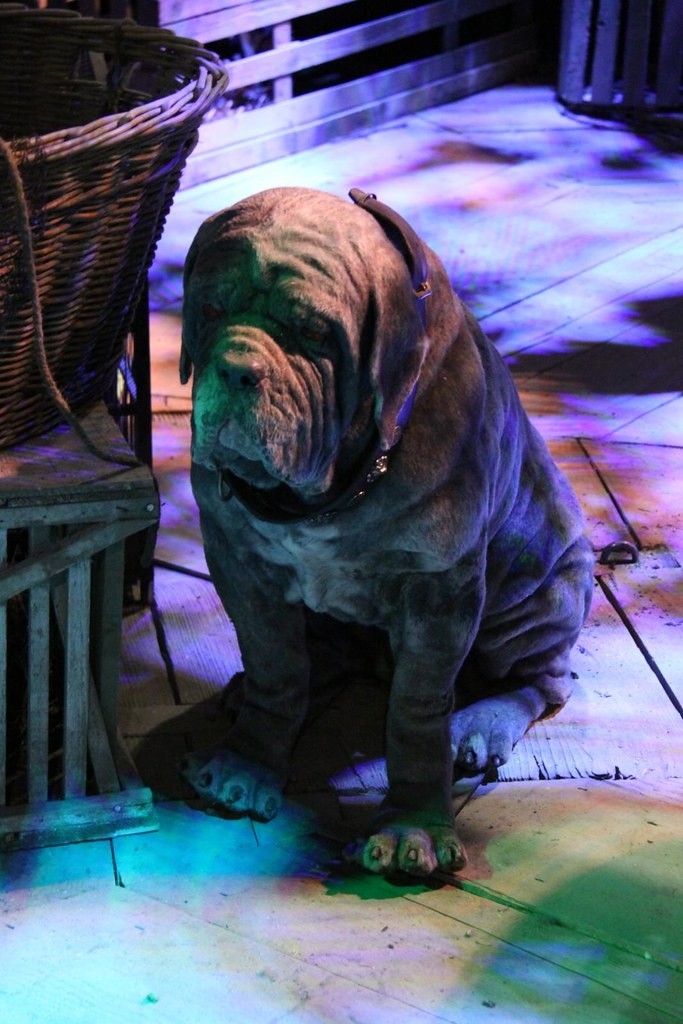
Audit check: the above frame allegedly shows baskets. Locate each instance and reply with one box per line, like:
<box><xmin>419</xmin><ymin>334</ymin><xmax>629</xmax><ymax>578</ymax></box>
<box><xmin>1</xmin><ymin>2</ymin><xmax>229</xmax><ymax>448</ymax></box>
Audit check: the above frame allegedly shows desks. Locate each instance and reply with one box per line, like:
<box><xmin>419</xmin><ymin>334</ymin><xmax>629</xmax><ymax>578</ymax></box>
<box><xmin>0</xmin><ymin>400</ymin><xmax>161</xmax><ymax>855</ymax></box>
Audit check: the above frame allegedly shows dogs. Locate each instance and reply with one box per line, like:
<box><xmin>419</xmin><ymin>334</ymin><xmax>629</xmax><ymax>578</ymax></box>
<box><xmin>119</xmin><ymin>185</ymin><xmax>592</xmax><ymax>872</ymax></box>
